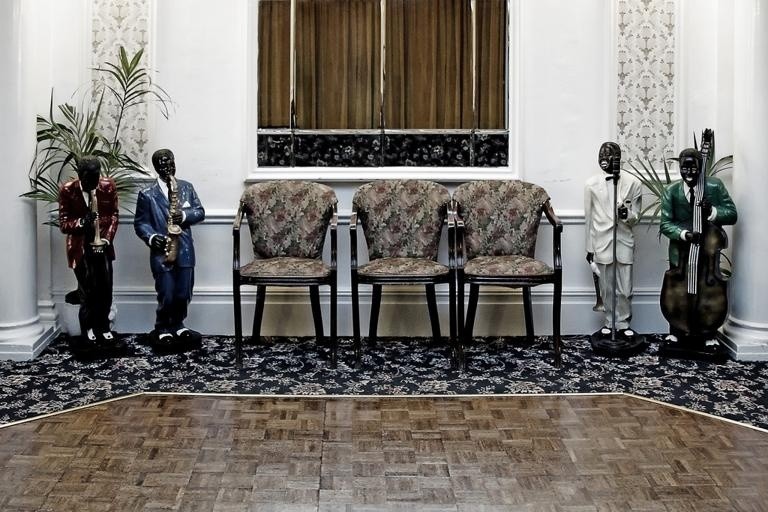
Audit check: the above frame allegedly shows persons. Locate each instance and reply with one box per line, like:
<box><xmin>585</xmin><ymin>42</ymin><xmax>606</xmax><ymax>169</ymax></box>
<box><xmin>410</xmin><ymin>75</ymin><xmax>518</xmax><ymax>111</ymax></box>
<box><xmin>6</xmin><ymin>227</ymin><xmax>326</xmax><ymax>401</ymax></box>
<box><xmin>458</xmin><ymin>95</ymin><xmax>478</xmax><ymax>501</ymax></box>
<box><xmin>134</xmin><ymin>148</ymin><xmax>206</xmax><ymax>345</ymax></box>
<box><xmin>658</xmin><ymin>149</ymin><xmax>739</xmax><ymax>353</ymax></box>
<box><xmin>57</xmin><ymin>155</ymin><xmax>121</xmax><ymax>345</ymax></box>
<box><xmin>583</xmin><ymin>142</ymin><xmax>644</xmax><ymax>345</ymax></box>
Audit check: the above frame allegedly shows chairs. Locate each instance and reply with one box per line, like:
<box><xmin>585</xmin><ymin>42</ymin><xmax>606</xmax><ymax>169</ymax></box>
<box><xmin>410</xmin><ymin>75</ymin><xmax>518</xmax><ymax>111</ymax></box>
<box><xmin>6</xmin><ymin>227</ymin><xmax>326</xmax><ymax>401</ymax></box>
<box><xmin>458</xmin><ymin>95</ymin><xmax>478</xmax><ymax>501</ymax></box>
<box><xmin>233</xmin><ymin>180</ymin><xmax>338</xmax><ymax>369</ymax></box>
<box><xmin>452</xmin><ymin>181</ymin><xmax>563</xmax><ymax>368</ymax></box>
<box><xmin>349</xmin><ymin>180</ymin><xmax>458</xmax><ymax>368</ymax></box>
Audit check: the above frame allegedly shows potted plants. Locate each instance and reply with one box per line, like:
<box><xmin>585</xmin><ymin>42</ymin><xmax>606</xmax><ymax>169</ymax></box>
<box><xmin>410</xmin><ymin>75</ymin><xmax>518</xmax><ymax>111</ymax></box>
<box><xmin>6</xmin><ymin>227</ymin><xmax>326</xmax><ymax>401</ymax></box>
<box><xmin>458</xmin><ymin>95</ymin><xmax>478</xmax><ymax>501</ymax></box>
<box><xmin>18</xmin><ymin>45</ymin><xmax>180</xmax><ymax>336</ymax></box>
